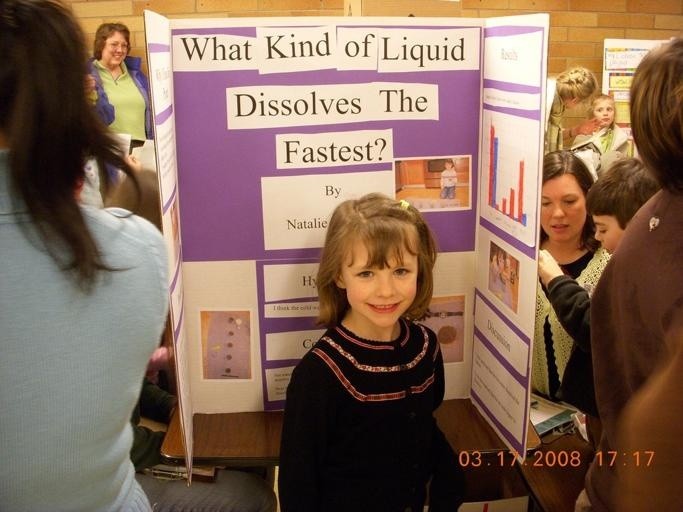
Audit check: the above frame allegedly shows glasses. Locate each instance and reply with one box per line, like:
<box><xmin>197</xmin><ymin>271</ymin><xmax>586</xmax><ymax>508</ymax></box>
<box><xmin>109</xmin><ymin>43</ymin><xmax>129</xmax><ymax>51</ymax></box>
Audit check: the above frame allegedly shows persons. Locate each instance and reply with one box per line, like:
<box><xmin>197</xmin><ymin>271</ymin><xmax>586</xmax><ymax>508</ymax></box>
<box><xmin>528</xmin><ymin>67</ymin><xmax>662</xmax><ymax>447</ymax></box>
<box><xmin>84</xmin><ymin>23</ymin><xmax>154</xmax><ymax>197</ymax></box>
<box><xmin>0</xmin><ymin>1</ymin><xmax>171</xmax><ymax>512</ymax></box>
<box><xmin>439</xmin><ymin>160</ymin><xmax>457</xmax><ymax>198</ymax></box>
<box><xmin>585</xmin><ymin>42</ymin><xmax>683</xmax><ymax>510</ymax></box>
<box><xmin>104</xmin><ymin>168</ymin><xmax>181</xmax><ymax>474</ymax></box>
<box><xmin>276</xmin><ymin>190</ymin><xmax>463</xmax><ymax>512</ymax></box>
